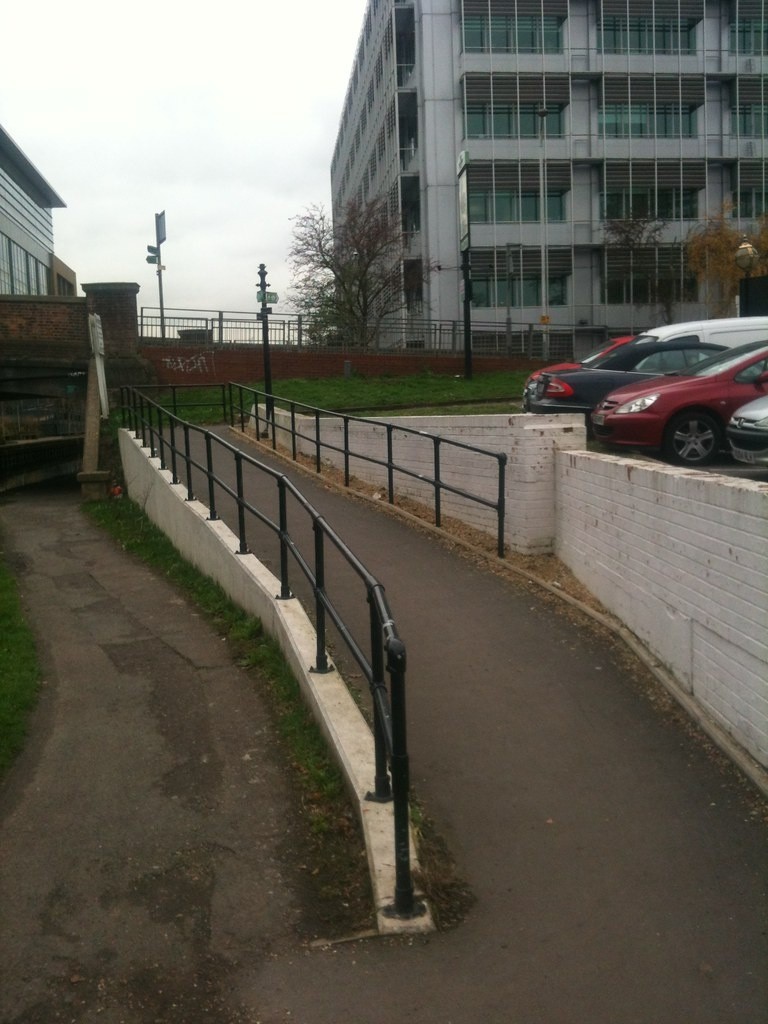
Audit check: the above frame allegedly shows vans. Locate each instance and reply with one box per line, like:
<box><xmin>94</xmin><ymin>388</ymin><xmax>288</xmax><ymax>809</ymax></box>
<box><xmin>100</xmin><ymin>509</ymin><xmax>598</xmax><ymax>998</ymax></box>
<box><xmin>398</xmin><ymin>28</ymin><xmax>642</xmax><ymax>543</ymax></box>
<box><xmin>635</xmin><ymin>317</ymin><xmax>768</xmax><ymax>378</ymax></box>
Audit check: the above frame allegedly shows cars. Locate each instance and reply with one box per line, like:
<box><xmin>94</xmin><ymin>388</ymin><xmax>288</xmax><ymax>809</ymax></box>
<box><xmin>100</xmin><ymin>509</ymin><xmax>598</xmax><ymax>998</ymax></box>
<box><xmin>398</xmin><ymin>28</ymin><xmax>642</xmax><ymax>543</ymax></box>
<box><xmin>591</xmin><ymin>340</ymin><xmax>768</xmax><ymax>467</ymax></box>
<box><xmin>525</xmin><ymin>334</ymin><xmax>637</xmax><ymax>388</ymax></box>
<box><xmin>729</xmin><ymin>394</ymin><xmax>768</xmax><ymax>472</ymax></box>
<box><xmin>527</xmin><ymin>341</ymin><xmax>736</xmax><ymax>441</ymax></box>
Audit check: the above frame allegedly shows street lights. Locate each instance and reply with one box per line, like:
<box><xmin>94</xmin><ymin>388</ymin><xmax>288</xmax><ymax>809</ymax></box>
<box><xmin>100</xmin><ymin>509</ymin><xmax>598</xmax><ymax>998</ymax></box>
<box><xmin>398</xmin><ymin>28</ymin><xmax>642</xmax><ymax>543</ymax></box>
<box><xmin>736</xmin><ymin>235</ymin><xmax>759</xmax><ymax>316</ymax></box>
